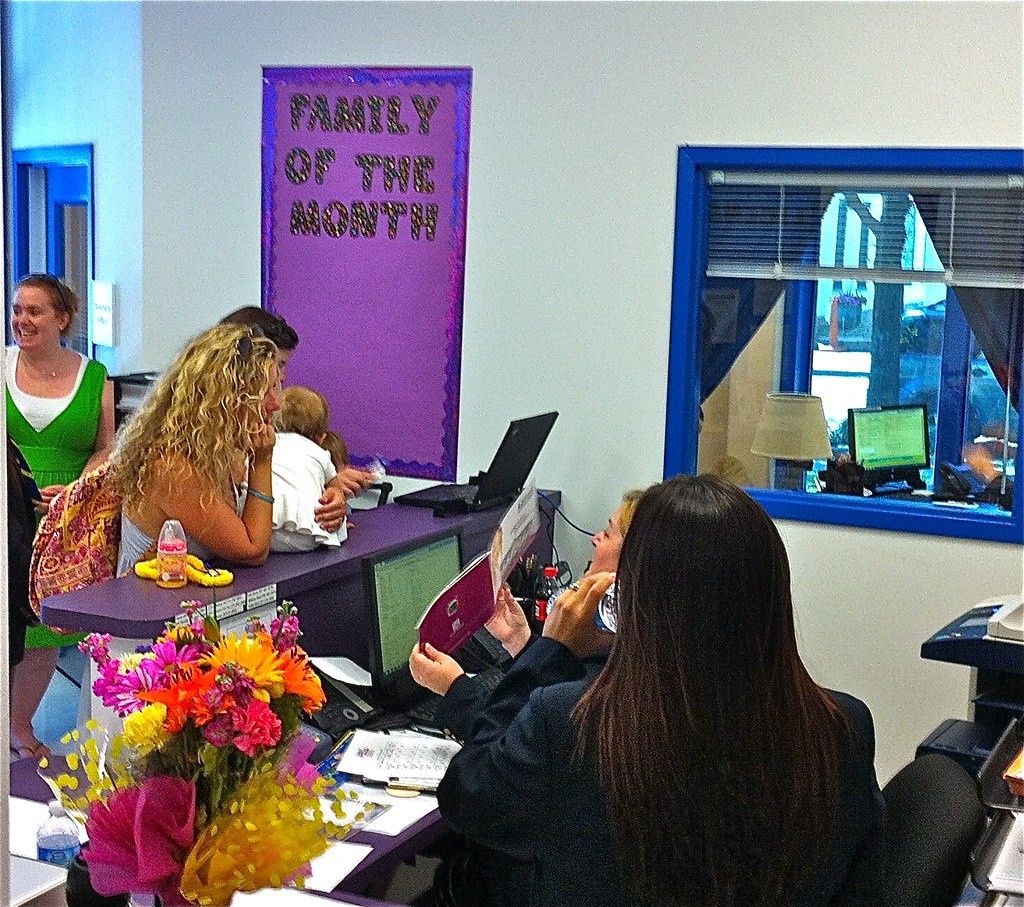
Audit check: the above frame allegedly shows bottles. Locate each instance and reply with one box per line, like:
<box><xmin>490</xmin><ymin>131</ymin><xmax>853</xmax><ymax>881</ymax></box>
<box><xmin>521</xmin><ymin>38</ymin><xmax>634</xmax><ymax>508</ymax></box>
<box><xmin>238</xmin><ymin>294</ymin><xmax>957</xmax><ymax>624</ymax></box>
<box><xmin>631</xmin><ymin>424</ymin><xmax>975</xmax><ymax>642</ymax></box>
<box><xmin>155</xmin><ymin>520</ymin><xmax>191</xmax><ymax>589</ymax></box>
<box><xmin>37</xmin><ymin>800</ymin><xmax>81</xmax><ymax>871</ymax></box>
<box><xmin>534</xmin><ymin>567</ymin><xmax>562</xmax><ymax>636</ymax></box>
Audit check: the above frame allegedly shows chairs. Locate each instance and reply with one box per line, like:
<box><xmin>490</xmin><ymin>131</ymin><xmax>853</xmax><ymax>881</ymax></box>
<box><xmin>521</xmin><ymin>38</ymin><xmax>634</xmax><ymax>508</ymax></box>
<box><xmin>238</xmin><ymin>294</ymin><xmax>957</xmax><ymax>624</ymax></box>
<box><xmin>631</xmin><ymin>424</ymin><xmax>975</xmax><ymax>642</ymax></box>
<box><xmin>825</xmin><ymin>750</ymin><xmax>987</xmax><ymax>907</ymax></box>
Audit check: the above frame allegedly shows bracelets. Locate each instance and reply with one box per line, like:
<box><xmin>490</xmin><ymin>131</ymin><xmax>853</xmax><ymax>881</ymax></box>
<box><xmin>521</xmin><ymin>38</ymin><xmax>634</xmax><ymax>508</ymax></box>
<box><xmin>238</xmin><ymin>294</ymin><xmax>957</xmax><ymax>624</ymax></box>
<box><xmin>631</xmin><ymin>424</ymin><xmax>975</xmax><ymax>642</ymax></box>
<box><xmin>247</xmin><ymin>489</ymin><xmax>274</xmax><ymax>503</ymax></box>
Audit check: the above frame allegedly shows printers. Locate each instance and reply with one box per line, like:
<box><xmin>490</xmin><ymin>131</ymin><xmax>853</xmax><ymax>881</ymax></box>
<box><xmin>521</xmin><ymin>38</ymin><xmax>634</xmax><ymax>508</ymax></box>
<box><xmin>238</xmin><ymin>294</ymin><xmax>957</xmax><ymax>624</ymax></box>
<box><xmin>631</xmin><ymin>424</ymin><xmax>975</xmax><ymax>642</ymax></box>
<box><xmin>920</xmin><ymin>592</ymin><xmax>1024</xmax><ymax>728</ymax></box>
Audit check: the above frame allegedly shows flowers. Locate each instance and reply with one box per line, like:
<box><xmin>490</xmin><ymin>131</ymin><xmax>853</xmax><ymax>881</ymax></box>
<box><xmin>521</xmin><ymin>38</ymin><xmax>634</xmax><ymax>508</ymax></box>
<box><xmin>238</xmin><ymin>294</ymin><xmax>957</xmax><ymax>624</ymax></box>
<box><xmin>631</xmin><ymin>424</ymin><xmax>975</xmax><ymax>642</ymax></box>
<box><xmin>37</xmin><ymin>598</ymin><xmax>378</xmax><ymax>907</ymax></box>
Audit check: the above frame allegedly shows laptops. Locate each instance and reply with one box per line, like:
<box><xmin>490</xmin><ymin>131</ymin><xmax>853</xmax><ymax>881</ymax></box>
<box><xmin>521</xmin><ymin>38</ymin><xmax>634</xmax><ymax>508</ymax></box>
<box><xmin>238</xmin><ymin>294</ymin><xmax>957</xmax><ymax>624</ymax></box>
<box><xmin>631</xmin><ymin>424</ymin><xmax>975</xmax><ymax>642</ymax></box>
<box><xmin>393</xmin><ymin>411</ymin><xmax>560</xmax><ymax>512</ymax></box>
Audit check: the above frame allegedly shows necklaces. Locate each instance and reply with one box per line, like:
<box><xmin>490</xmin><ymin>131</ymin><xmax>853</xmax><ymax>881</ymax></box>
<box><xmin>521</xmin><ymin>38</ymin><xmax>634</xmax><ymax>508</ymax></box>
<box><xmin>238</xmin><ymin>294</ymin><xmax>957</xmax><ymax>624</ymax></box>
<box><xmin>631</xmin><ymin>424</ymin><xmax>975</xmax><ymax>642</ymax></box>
<box><xmin>22</xmin><ymin>354</ymin><xmax>58</xmax><ymax>378</ymax></box>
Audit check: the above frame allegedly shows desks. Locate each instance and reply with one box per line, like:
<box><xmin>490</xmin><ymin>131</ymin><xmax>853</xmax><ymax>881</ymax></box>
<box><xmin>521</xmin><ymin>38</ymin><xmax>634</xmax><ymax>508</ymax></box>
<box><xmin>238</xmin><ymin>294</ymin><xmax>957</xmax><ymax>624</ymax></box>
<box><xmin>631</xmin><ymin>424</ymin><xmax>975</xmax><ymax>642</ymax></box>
<box><xmin>6</xmin><ymin>488</ymin><xmax>563</xmax><ymax>907</ymax></box>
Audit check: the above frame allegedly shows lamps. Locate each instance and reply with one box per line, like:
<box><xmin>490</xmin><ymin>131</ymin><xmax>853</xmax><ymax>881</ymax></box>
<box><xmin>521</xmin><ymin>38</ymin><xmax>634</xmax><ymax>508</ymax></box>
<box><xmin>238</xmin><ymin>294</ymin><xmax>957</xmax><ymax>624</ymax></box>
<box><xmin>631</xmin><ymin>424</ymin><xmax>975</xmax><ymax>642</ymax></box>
<box><xmin>749</xmin><ymin>392</ymin><xmax>833</xmax><ymax>492</ymax></box>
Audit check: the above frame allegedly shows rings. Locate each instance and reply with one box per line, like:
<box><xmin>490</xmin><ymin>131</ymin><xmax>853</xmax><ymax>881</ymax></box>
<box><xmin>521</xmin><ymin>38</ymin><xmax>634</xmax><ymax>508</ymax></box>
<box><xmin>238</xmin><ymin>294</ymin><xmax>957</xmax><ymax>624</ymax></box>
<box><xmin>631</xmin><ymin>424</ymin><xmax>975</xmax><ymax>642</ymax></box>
<box><xmin>569</xmin><ymin>582</ymin><xmax>580</xmax><ymax>590</ymax></box>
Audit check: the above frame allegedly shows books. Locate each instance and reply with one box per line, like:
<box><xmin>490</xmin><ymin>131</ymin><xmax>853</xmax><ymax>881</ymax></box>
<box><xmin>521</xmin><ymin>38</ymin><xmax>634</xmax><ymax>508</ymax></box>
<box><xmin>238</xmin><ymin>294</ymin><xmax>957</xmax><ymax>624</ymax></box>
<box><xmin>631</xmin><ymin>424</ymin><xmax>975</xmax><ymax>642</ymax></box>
<box><xmin>414</xmin><ymin>475</ymin><xmax>543</xmax><ymax>662</ymax></box>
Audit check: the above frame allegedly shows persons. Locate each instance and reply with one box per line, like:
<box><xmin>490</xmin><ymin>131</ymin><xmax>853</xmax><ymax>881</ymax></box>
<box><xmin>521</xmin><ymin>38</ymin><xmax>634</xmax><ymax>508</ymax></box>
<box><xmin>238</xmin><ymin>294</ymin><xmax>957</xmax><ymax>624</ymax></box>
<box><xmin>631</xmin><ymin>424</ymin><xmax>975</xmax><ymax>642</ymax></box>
<box><xmin>490</xmin><ymin>528</ymin><xmax>503</xmax><ymax>600</ymax></box>
<box><xmin>74</xmin><ymin>305</ymin><xmax>381</xmax><ymax>760</ymax></box>
<box><xmin>963</xmin><ymin>419</ymin><xmax>1018</xmax><ymax>513</ymax></box>
<box><xmin>8</xmin><ymin>273</ymin><xmax>116</xmax><ymax>759</ymax></box>
<box><xmin>434</xmin><ymin>470</ymin><xmax>889</xmax><ymax>907</ymax></box>
<box><xmin>406</xmin><ymin>488</ymin><xmax>647</xmax><ymax>747</ymax></box>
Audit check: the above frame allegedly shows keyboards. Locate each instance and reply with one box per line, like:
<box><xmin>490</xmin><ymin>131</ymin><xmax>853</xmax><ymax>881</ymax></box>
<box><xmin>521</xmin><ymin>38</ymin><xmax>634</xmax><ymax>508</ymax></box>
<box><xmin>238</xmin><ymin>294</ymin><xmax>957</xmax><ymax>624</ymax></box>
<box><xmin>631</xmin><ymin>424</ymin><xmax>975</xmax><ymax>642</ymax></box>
<box><xmin>405</xmin><ymin>668</ymin><xmax>506</xmax><ymax>726</ymax></box>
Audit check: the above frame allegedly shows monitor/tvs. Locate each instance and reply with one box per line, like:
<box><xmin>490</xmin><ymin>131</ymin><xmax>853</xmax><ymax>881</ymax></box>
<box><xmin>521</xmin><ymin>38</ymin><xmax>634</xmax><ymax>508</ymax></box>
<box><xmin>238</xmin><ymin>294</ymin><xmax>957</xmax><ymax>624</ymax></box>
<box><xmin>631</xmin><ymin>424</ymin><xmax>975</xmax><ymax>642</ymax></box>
<box><xmin>849</xmin><ymin>405</ymin><xmax>930</xmax><ymax>492</ymax></box>
<box><xmin>361</xmin><ymin>523</ymin><xmax>467</xmax><ymax>708</ymax></box>
